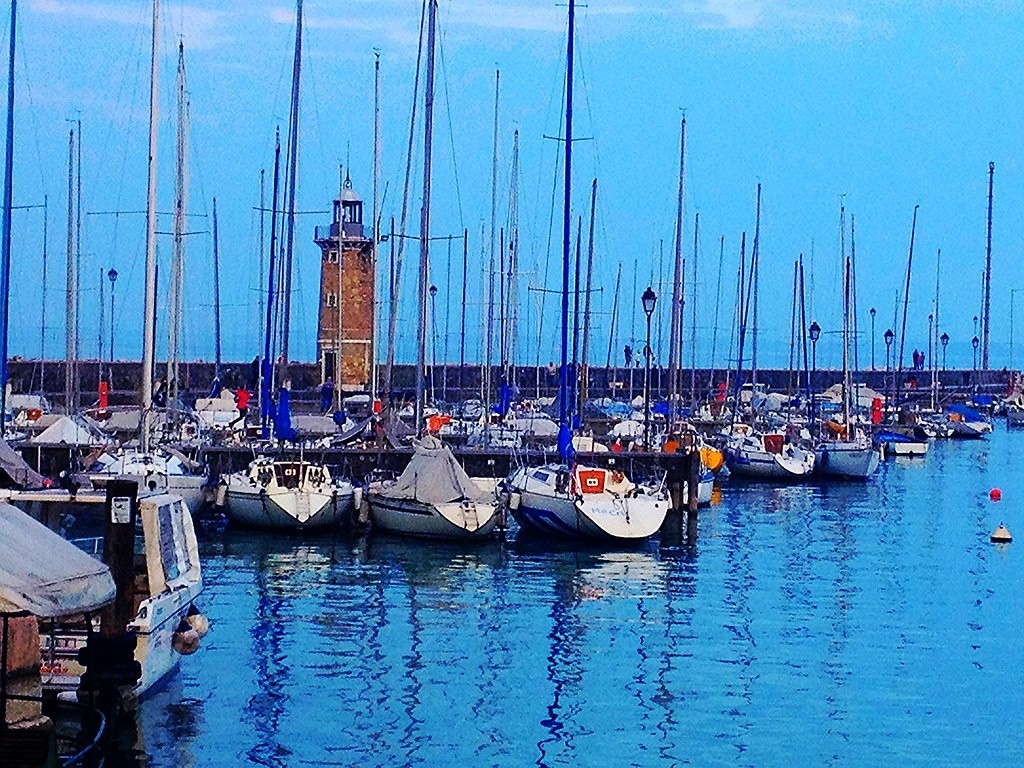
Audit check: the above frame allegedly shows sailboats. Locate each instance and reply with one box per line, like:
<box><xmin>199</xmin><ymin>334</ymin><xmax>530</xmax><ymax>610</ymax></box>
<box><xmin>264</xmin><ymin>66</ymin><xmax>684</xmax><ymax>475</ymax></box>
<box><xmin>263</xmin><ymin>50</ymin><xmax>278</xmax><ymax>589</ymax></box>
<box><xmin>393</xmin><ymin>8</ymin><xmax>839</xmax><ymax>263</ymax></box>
<box><xmin>2</xmin><ymin>0</ymin><xmax>1023</xmax><ymax>717</ymax></box>
